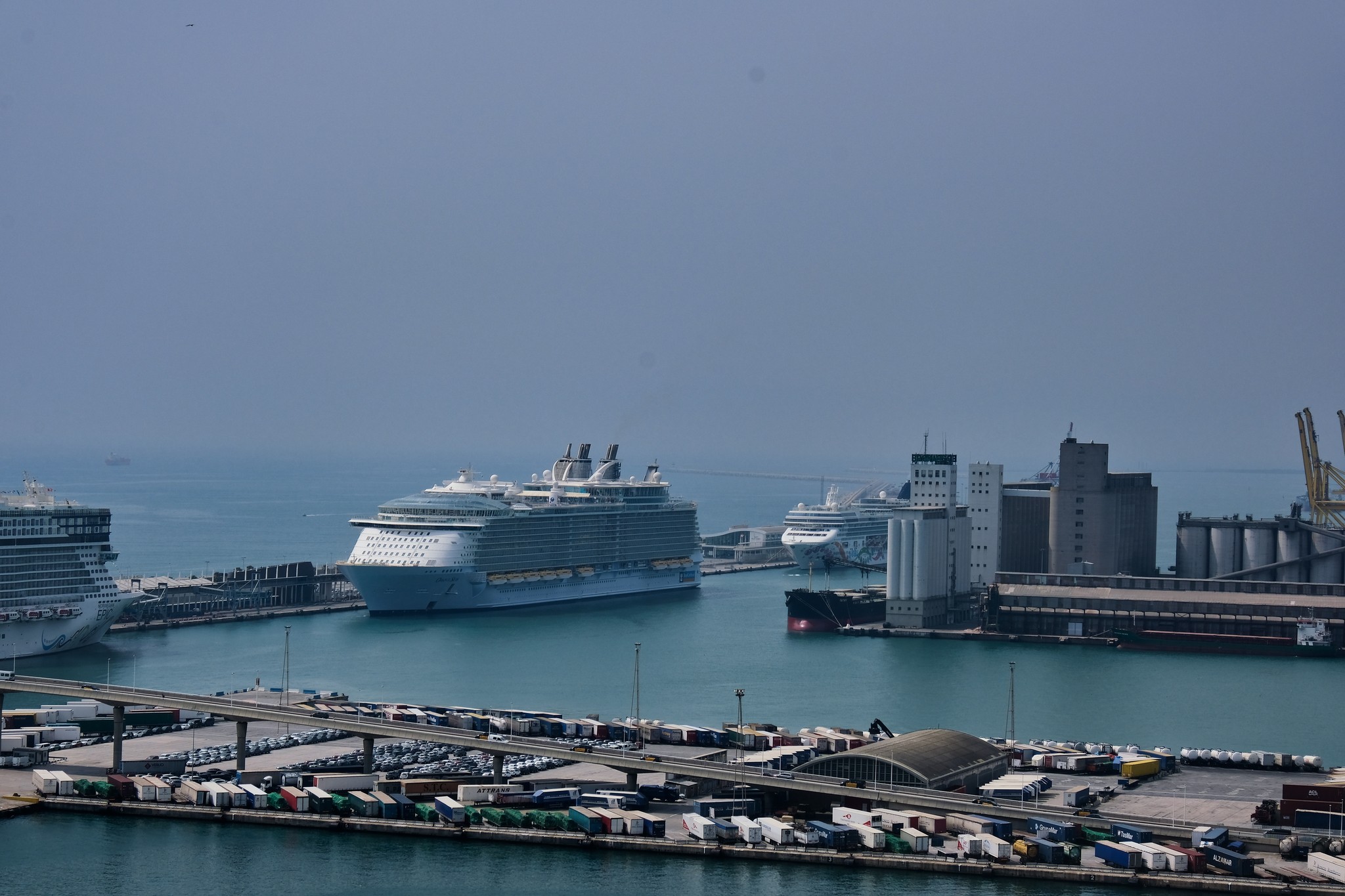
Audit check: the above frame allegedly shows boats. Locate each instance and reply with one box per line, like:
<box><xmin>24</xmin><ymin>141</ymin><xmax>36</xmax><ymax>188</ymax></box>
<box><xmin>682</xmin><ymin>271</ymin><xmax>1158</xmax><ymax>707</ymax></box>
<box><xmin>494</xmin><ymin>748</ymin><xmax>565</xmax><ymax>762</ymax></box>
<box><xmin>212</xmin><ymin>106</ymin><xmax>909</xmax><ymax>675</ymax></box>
<box><xmin>0</xmin><ymin>605</ymin><xmax>84</xmax><ymax>625</ymax></box>
<box><xmin>650</xmin><ymin>556</ymin><xmax>695</xmax><ymax>571</ymax></box>
<box><xmin>783</xmin><ymin>555</ymin><xmax>885</xmax><ymax>634</ymax></box>
<box><xmin>487</xmin><ymin>567</ymin><xmax>595</xmax><ymax>586</ymax></box>
<box><xmin>1110</xmin><ymin>602</ymin><xmax>1340</xmax><ymax>659</ymax></box>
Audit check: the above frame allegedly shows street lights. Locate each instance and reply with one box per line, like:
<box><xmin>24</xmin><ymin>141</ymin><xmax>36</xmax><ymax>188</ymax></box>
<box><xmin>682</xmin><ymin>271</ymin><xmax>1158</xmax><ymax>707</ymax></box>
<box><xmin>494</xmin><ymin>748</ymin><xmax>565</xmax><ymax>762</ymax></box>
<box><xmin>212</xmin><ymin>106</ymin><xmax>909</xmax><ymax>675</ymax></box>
<box><xmin>13</xmin><ymin>643</ymin><xmax>16</xmax><ymax>674</ymax></box>
<box><xmin>488</xmin><ymin>706</ymin><xmax>492</xmax><ymax>742</ymax></box>
<box><xmin>133</xmin><ymin>655</ymin><xmax>136</xmax><ymax>693</ymax></box>
<box><xmin>256</xmin><ymin>670</ymin><xmax>259</xmax><ymax>708</ymax></box>
<box><xmin>381</xmin><ymin>686</ymin><xmax>384</xmax><ymax>724</ymax></box>
<box><xmin>107</xmin><ymin>658</ymin><xmax>110</xmax><ymax>692</ymax></box>
<box><xmin>231</xmin><ymin>672</ymin><xmax>234</xmax><ymax>707</ymax></box>
<box><xmin>358</xmin><ymin>689</ymin><xmax>361</xmax><ymax>723</ymax></box>
<box><xmin>510</xmin><ymin>702</ymin><xmax>513</xmax><ymax>741</ymax></box>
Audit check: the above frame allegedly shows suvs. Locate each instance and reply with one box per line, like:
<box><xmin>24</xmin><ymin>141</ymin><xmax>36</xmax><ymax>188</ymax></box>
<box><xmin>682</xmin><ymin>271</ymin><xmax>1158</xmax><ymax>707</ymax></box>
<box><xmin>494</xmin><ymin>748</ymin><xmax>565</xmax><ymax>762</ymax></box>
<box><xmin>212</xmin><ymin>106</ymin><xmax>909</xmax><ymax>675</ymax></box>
<box><xmin>475</xmin><ymin>732</ymin><xmax>498</xmax><ymax>742</ymax></box>
<box><xmin>309</xmin><ymin>711</ymin><xmax>329</xmax><ymax>719</ymax></box>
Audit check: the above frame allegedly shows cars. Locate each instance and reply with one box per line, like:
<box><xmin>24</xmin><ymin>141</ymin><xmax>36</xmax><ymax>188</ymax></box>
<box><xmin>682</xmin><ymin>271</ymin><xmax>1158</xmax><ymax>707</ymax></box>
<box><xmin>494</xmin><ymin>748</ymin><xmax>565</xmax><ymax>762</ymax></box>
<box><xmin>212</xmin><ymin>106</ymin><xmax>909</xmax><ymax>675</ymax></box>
<box><xmin>30</xmin><ymin>716</ymin><xmax>574</xmax><ymax>788</ymax></box>
<box><xmin>488</xmin><ymin>734</ymin><xmax>509</xmax><ymax>744</ymax></box>
<box><xmin>80</xmin><ymin>684</ymin><xmax>100</xmax><ymax>692</ymax></box>
<box><xmin>547</xmin><ymin>736</ymin><xmax>645</xmax><ymax>754</ymax></box>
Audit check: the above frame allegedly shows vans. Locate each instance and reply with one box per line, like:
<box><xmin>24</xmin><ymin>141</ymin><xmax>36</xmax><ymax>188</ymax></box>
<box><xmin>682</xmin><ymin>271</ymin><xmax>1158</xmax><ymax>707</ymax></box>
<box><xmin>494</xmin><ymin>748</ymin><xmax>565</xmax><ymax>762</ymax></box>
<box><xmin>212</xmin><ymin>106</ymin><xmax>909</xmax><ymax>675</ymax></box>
<box><xmin>0</xmin><ymin>670</ymin><xmax>15</xmax><ymax>681</ymax></box>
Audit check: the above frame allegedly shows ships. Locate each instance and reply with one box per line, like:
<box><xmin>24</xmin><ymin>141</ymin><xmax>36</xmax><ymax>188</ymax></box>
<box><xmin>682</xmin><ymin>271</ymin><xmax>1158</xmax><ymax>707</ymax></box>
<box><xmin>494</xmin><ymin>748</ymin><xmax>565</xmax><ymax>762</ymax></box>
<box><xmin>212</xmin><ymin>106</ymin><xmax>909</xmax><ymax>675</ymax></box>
<box><xmin>334</xmin><ymin>440</ymin><xmax>706</xmax><ymax>617</ymax></box>
<box><xmin>780</xmin><ymin>475</ymin><xmax>908</xmax><ymax>570</ymax></box>
<box><xmin>0</xmin><ymin>471</ymin><xmax>146</xmax><ymax>663</ymax></box>
